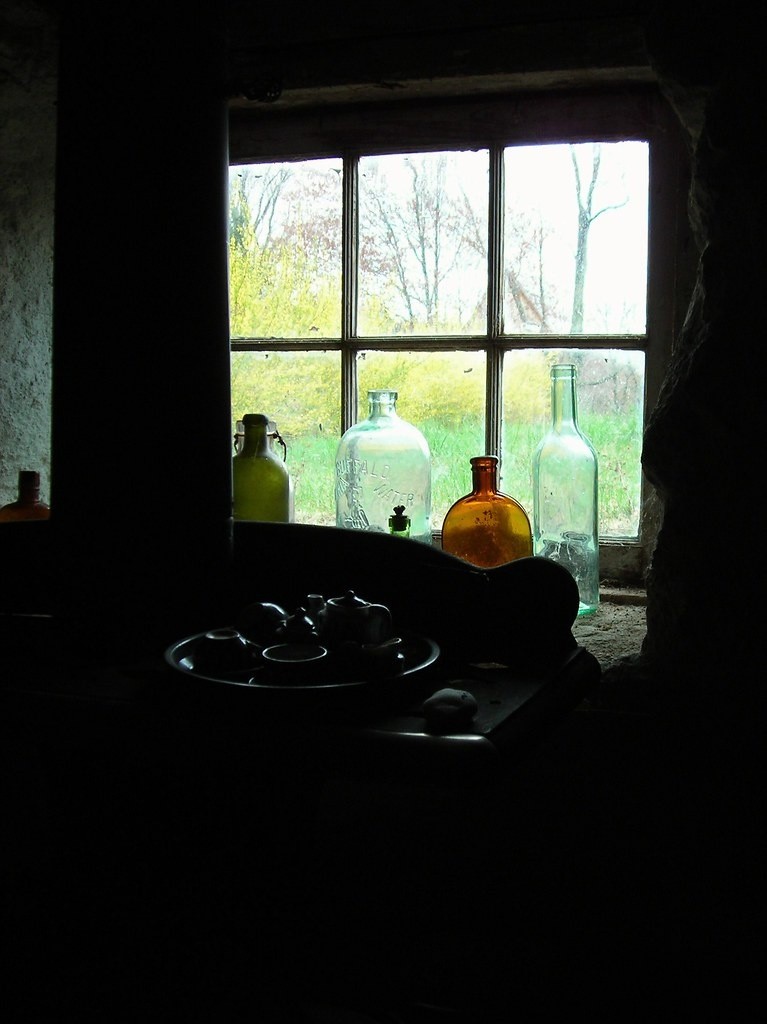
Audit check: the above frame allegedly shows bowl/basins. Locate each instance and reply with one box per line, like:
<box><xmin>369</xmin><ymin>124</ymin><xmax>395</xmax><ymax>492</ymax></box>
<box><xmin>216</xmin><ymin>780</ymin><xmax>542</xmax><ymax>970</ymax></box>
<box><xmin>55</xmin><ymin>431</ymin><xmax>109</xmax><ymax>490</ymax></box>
<box><xmin>263</xmin><ymin>644</ymin><xmax>327</xmax><ymax>685</ymax></box>
<box><xmin>195</xmin><ymin>630</ymin><xmax>259</xmax><ymax>679</ymax></box>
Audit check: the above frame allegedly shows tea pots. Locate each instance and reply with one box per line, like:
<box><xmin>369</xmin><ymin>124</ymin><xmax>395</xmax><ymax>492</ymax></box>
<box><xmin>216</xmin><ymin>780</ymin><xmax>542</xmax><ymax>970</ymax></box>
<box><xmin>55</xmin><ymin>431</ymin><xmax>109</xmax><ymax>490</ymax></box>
<box><xmin>275</xmin><ymin>607</ymin><xmax>321</xmax><ymax>645</ymax></box>
<box><xmin>306</xmin><ymin>591</ymin><xmax>389</xmax><ymax>651</ymax></box>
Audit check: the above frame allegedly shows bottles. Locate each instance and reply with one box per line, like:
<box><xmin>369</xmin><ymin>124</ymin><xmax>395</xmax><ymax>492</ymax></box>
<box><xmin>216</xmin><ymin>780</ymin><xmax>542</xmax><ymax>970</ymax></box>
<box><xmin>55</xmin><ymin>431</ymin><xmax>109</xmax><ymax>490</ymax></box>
<box><xmin>229</xmin><ymin>413</ymin><xmax>295</xmax><ymax>528</ymax></box>
<box><xmin>530</xmin><ymin>361</ymin><xmax>601</xmax><ymax>617</ymax></box>
<box><xmin>2</xmin><ymin>467</ymin><xmax>47</xmax><ymax>525</ymax></box>
<box><xmin>440</xmin><ymin>455</ymin><xmax>530</xmax><ymax>570</ymax></box>
<box><xmin>331</xmin><ymin>389</ymin><xmax>433</xmax><ymax>553</ymax></box>
